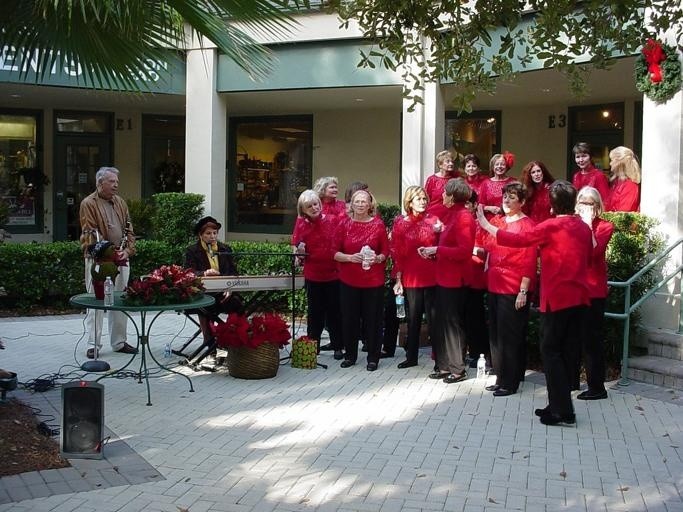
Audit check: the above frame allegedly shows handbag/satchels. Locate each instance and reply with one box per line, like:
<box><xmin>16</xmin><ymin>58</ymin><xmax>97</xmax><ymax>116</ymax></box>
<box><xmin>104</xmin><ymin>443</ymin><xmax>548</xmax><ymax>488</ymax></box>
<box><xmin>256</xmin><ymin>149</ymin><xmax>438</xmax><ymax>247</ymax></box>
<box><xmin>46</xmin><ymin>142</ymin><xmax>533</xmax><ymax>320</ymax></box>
<box><xmin>292</xmin><ymin>335</ymin><xmax>317</xmax><ymax>369</ymax></box>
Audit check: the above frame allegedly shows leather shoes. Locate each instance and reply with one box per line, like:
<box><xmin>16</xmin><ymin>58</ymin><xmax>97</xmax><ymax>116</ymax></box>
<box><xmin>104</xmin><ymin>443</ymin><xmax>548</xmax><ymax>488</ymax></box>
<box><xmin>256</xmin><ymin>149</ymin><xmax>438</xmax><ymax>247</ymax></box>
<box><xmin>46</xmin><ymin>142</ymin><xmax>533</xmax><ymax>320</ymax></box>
<box><xmin>113</xmin><ymin>343</ymin><xmax>139</xmax><ymax>353</ymax></box>
<box><xmin>87</xmin><ymin>348</ymin><xmax>99</xmax><ymax>359</ymax></box>
<box><xmin>317</xmin><ymin>343</ymin><xmax>469</xmax><ymax>384</ymax></box>
<box><xmin>469</xmin><ymin>359</ymin><xmax>608</xmax><ymax>425</ymax></box>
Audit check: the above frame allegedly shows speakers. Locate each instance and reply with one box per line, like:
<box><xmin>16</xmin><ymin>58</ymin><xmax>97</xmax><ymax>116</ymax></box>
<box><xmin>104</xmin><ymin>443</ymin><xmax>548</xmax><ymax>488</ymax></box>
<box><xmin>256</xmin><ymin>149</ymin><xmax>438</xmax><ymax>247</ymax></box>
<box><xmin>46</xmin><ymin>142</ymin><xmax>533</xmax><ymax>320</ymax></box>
<box><xmin>59</xmin><ymin>381</ymin><xmax>104</xmax><ymax>460</ymax></box>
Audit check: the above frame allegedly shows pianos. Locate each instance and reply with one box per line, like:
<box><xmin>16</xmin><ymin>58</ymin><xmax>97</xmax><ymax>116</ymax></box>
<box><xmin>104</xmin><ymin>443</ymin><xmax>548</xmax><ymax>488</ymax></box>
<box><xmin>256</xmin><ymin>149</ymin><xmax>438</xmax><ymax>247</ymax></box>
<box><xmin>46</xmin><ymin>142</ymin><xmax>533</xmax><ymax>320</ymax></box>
<box><xmin>198</xmin><ymin>275</ymin><xmax>305</xmax><ymax>292</ymax></box>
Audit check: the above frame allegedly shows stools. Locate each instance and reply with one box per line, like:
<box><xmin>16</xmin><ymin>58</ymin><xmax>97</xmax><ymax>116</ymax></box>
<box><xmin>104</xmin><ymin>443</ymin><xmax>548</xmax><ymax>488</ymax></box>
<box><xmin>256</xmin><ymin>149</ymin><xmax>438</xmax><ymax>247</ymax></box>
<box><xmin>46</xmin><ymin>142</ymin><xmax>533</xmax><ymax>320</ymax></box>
<box><xmin>171</xmin><ymin>306</ymin><xmax>202</xmax><ymax>356</ymax></box>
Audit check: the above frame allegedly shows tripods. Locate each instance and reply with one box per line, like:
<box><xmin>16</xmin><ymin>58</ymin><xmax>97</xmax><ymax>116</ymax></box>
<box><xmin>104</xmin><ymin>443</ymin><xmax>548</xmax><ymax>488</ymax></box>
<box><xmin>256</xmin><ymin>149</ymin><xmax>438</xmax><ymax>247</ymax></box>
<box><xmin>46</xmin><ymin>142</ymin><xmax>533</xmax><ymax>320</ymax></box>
<box><xmin>276</xmin><ymin>257</ymin><xmax>329</xmax><ymax>370</ymax></box>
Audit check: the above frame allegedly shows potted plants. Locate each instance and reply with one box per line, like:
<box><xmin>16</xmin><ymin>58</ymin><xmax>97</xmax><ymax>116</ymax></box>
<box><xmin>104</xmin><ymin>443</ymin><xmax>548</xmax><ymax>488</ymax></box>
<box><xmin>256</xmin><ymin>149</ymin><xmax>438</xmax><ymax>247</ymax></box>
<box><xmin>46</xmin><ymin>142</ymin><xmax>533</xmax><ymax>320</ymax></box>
<box><xmin>90</xmin><ymin>262</ymin><xmax>118</xmax><ymax>300</ymax></box>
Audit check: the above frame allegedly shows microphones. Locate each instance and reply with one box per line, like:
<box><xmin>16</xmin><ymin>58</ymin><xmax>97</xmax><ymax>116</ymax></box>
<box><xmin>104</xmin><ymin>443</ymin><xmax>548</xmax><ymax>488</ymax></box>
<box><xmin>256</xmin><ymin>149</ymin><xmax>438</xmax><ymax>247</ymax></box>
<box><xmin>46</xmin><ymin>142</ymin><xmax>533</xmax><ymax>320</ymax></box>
<box><xmin>205</xmin><ymin>240</ymin><xmax>214</xmax><ymax>258</ymax></box>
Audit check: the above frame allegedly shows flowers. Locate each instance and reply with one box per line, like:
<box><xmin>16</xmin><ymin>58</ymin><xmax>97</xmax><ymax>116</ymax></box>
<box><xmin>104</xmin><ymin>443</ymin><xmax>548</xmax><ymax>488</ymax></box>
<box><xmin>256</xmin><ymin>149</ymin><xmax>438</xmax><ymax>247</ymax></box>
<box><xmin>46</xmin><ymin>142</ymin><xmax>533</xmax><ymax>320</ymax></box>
<box><xmin>88</xmin><ymin>239</ymin><xmax>108</xmax><ymax>256</ymax></box>
<box><xmin>119</xmin><ymin>264</ymin><xmax>208</xmax><ymax>306</ymax></box>
<box><xmin>209</xmin><ymin>310</ymin><xmax>294</xmax><ymax>350</ymax></box>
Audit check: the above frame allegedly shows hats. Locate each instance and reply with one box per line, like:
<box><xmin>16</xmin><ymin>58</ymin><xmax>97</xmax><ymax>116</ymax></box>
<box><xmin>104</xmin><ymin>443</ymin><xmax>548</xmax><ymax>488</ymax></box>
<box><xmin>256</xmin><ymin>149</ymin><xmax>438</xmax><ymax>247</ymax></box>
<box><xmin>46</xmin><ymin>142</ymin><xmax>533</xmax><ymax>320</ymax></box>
<box><xmin>194</xmin><ymin>217</ymin><xmax>221</xmax><ymax>236</ymax></box>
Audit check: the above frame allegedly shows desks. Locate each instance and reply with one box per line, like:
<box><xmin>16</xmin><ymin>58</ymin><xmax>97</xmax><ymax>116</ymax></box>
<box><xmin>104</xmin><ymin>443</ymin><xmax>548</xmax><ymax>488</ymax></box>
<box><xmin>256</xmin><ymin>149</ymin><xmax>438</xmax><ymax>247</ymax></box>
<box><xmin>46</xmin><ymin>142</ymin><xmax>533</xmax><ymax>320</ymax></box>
<box><xmin>70</xmin><ymin>289</ymin><xmax>214</xmax><ymax>407</ymax></box>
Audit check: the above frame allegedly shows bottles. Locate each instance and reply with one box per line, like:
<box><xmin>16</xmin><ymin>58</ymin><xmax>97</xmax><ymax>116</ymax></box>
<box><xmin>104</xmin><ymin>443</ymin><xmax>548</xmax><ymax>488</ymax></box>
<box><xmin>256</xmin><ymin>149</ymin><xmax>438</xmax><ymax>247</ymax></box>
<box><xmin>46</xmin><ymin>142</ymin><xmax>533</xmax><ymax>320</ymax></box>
<box><xmin>103</xmin><ymin>276</ymin><xmax>114</xmax><ymax>307</ymax></box>
<box><xmin>361</xmin><ymin>245</ymin><xmax>371</xmax><ymax>270</ymax></box>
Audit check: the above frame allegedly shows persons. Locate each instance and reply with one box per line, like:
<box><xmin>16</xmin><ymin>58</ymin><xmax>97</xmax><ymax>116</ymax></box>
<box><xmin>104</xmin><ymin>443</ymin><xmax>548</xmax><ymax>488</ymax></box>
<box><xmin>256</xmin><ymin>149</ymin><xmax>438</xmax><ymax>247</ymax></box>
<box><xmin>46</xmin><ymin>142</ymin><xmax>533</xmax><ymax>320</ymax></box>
<box><xmin>185</xmin><ymin>216</ymin><xmax>244</xmax><ymax>357</ymax></box>
<box><xmin>292</xmin><ymin>142</ymin><xmax>641</xmax><ymax>401</ymax></box>
<box><xmin>78</xmin><ymin>165</ymin><xmax>138</xmax><ymax>359</ymax></box>
<box><xmin>476</xmin><ymin>178</ymin><xmax>594</xmax><ymax>425</ymax></box>
